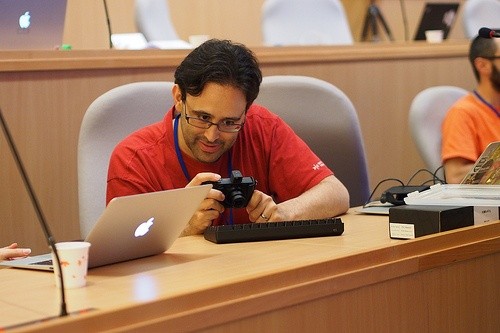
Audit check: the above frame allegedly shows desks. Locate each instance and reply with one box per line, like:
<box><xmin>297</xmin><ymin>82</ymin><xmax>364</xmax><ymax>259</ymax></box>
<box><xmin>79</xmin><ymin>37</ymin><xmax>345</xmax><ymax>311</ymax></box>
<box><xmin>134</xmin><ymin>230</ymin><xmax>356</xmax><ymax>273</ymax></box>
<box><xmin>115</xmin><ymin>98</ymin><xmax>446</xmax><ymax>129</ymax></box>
<box><xmin>0</xmin><ymin>201</ymin><xmax>500</xmax><ymax>333</ymax></box>
<box><xmin>0</xmin><ymin>36</ymin><xmax>479</xmax><ymax>257</ymax></box>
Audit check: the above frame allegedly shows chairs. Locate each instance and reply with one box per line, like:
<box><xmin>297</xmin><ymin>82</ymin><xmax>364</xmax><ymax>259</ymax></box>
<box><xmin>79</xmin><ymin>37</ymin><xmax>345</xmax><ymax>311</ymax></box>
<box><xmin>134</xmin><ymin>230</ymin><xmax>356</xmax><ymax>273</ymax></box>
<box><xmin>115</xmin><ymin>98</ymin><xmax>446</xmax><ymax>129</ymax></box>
<box><xmin>262</xmin><ymin>0</ymin><xmax>355</xmax><ymax>46</ymax></box>
<box><xmin>409</xmin><ymin>84</ymin><xmax>471</xmax><ymax>183</ymax></box>
<box><xmin>76</xmin><ymin>81</ymin><xmax>177</xmax><ymax>242</ymax></box>
<box><xmin>461</xmin><ymin>0</ymin><xmax>500</xmax><ymax>41</ymax></box>
<box><xmin>254</xmin><ymin>74</ymin><xmax>372</xmax><ymax>208</ymax></box>
<box><xmin>134</xmin><ymin>0</ymin><xmax>181</xmax><ymax>42</ymax></box>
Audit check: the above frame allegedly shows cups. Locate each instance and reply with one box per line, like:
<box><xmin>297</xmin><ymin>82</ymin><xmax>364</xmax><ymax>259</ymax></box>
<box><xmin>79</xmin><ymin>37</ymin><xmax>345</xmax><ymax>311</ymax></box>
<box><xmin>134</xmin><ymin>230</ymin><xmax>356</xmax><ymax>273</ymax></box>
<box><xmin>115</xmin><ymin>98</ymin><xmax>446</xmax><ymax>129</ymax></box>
<box><xmin>49</xmin><ymin>241</ymin><xmax>92</xmax><ymax>286</ymax></box>
<box><xmin>424</xmin><ymin>29</ymin><xmax>444</xmax><ymax>43</ymax></box>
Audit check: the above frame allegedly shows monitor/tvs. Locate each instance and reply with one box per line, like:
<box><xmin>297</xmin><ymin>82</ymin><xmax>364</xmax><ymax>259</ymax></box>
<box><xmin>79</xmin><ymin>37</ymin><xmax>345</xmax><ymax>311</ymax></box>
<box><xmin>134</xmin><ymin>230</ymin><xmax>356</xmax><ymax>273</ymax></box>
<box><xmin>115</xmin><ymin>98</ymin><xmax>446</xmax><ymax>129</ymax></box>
<box><xmin>414</xmin><ymin>2</ymin><xmax>458</xmax><ymax>40</ymax></box>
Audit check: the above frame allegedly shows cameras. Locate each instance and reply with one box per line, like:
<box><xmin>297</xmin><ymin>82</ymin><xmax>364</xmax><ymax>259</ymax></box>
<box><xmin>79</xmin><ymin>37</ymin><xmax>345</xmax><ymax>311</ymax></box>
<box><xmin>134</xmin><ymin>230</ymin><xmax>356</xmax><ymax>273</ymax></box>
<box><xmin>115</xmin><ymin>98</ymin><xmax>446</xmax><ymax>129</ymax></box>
<box><xmin>203</xmin><ymin>169</ymin><xmax>257</xmax><ymax>209</ymax></box>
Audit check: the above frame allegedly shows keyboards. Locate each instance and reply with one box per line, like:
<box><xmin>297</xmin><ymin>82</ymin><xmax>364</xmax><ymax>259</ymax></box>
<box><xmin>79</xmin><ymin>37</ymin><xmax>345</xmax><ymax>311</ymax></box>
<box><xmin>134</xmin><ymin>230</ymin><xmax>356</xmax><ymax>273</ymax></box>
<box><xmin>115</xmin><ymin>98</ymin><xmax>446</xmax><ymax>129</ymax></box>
<box><xmin>204</xmin><ymin>217</ymin><xmax>344</xmax><ymax>243</ymax></box>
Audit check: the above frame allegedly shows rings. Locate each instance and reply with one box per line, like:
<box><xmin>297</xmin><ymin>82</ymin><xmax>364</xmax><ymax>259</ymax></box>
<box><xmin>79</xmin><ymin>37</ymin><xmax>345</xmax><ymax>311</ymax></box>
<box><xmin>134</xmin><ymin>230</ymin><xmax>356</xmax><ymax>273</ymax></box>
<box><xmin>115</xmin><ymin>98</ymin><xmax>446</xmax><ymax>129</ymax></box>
<box><xmin>261</xmin><ymin>214</ymin><xmax>267</xmax><ymax>218</ymax></box>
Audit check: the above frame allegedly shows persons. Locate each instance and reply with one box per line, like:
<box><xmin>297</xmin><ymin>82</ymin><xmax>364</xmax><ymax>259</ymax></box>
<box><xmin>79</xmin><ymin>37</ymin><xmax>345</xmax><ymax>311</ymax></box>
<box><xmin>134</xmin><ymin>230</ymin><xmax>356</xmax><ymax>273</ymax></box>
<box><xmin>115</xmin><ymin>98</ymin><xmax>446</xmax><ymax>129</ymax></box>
<box><xmin>441</xmin><ymin>28</ymin><xmax>500</xmax><ymax>184</ymax></box>
<box><xmin>0</xmin><ymin>243</ymin><xmax>31</xmax><ymax>262</ymax></box>
<box><xmin>106</xmin><ymin>37</ymin><xmax>350</xmax><ymax>237</ymax></box>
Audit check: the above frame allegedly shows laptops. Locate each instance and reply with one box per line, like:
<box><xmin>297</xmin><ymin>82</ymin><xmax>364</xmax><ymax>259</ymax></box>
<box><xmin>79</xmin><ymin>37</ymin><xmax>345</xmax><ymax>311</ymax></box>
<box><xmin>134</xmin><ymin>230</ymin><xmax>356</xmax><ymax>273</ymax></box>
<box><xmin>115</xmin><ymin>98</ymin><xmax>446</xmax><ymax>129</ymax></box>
<box><xmin>0</xmin><ymin>184</ymin><xmax>211</xmax><ymax>271</ymax></box>
<box><xmin>0</xmin><ymin>0</ymin><xmax>68</xmax><ymax>51</ymax></box>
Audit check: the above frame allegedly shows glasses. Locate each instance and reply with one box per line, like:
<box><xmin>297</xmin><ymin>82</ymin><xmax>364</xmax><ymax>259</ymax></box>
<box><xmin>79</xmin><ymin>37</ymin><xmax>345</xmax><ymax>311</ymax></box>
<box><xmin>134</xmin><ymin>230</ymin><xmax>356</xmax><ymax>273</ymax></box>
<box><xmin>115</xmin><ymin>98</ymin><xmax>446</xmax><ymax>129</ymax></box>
<box><xmin>185</xmin><ymin>102</ymin><xmax>245</xmax><ymax>132</ymax></box>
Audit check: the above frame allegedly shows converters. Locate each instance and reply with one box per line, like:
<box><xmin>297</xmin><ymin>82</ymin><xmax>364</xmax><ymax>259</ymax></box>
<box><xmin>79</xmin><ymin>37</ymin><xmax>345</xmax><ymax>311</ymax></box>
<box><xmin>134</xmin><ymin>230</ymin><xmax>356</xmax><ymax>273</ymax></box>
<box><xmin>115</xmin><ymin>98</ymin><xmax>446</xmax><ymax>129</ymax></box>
<box><xmin>385</xmin><ymin>184</ymin><xmax>430</xmax><ymax>201</ymax></box>
